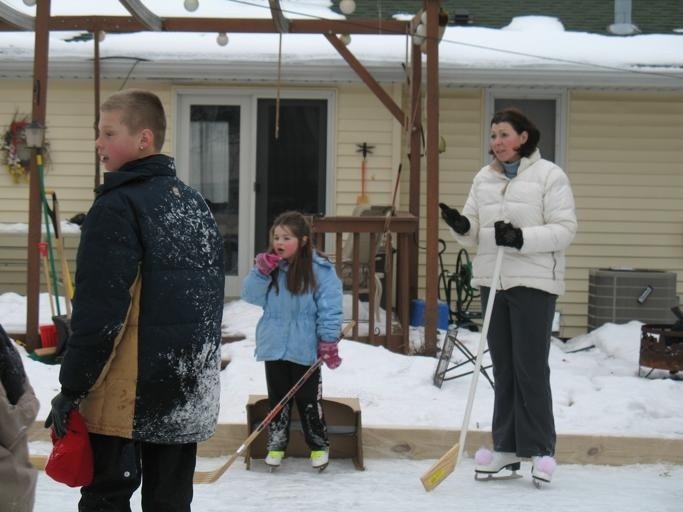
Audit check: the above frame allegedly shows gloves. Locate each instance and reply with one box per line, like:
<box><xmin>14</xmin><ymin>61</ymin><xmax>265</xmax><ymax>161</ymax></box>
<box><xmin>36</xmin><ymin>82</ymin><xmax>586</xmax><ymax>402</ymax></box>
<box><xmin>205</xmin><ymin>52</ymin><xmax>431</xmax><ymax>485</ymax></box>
<box><xmin>494</xmin><ymin>221</ymin><xmax>524</xmax><ymax>250</ymax></box>
<box><xmin>319</xmin><ymin>339</ymin><xmax>342</xmax><ymax>369</ymax></box>
<box><xmin>255</xmin><ymin>252</ymin><xmax>282</xmax><ymax>275</ymax></box>
<box><xmin>438</xmin><ymin>202</ymin><xmax>471</xmax><ymax>236</ymax></box>
<box><xmin>44</xmin><ymin>392</ymin><xmax>73</xmax><ymax>438</ymax></box>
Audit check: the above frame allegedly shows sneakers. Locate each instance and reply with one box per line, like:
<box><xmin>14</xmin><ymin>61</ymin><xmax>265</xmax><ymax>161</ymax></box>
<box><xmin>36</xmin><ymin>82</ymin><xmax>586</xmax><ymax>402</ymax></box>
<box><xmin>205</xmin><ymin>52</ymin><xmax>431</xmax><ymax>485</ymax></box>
<box><xmin>310</xmin><ymin>448</ymin><xmax>329</xmax><ymax>468</ymax></box>
<box><xmin>532</xmin><ymin>455</ymin><xmax>552</xmax><ymax>482</ymax></box>
<box><xmin>264</xmin><ymin>451</ymin><xmax>285</xmax><ymax>466</ymax></box>
<box><xmin>475</xmin><ymin>452</ymin><xmax>520</xmax><ymax>474</ymax></box>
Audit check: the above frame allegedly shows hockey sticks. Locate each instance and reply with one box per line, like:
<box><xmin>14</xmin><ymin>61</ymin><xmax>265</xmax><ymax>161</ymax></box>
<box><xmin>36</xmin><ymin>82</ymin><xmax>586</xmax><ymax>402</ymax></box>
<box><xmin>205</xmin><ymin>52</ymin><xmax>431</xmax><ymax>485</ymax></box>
<box><xmin>195</xmin><ymin>319</ymin><xmax>355</xmax><ymax>483</ymax></box>
<box><xmin>421</xmin><ymin>217</ymin><xmax>508</xmax><ymax>492</ymax></box>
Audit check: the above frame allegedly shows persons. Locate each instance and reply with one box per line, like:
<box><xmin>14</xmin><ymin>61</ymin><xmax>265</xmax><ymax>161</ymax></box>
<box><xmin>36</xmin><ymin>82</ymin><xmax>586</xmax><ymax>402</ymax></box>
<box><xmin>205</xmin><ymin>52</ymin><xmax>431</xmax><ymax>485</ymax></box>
<box><xmin>238</xmin><ymin>207</ymin><xmax>344</xmax><ymax>469</ymax></box>
<box><xmin>436</xmin><ymin>105</ymin><xmax>579</xmax><ymax>484</ymax></box>
<box><xmin>41</xmin><ymin>86</ymin><xmax>226</xmax><ymax>511</ymax></box>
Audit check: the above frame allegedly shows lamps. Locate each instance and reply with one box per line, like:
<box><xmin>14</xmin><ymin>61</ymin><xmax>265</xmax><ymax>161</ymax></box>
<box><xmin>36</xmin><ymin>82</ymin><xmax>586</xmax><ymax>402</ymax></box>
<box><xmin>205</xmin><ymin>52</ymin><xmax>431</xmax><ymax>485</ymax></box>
<box><xmin>340</xmin><ymin>0</ymin><xmax>356</xmax><ymax>14</ymax></box>
<box><xmin>340</xmin><ymin>35</ymin><xmax>353</xmax><ymax>43</ymax></box>
<box><xmin>218</xmin><ymin>33</ymin><xmax>229</xmax><ymax>46</ymax></box>
<box><xmin>184</xmin><ymin>0</ymin><xmax>199</xmax><ymax>12</ymax></box>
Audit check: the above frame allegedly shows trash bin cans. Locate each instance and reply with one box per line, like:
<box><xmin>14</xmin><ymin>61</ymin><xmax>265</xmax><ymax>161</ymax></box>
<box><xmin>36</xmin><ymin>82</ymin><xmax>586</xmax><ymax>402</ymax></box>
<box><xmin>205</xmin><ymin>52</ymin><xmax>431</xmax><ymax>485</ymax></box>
<box><xmin>639</xmin><ymin>325</ymin><xmax>683</xmax><ymax>380</ymax></box>
